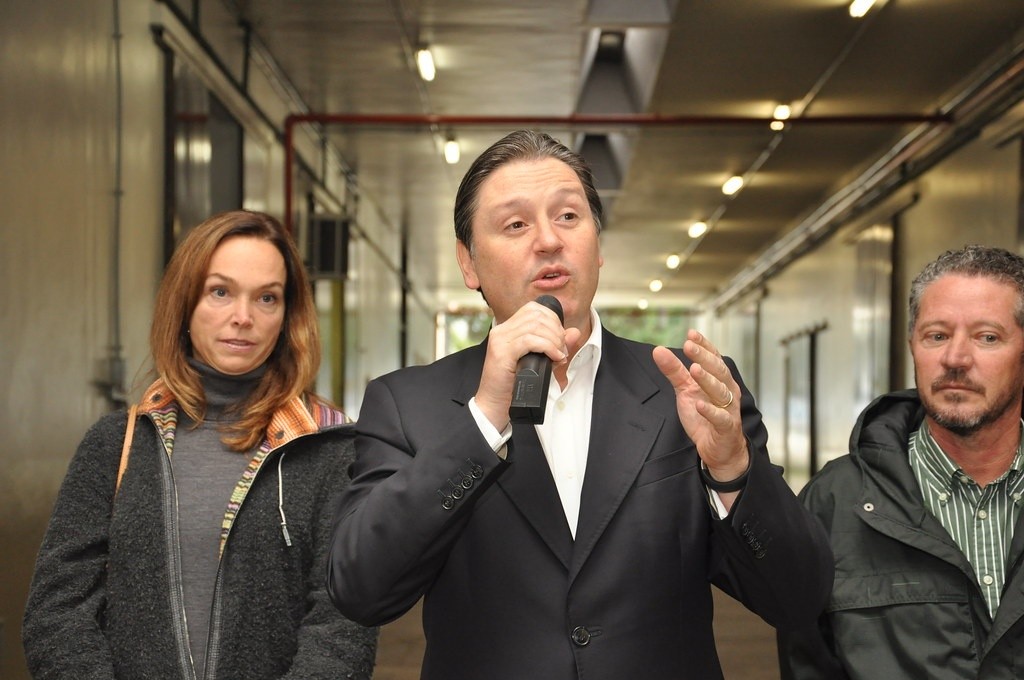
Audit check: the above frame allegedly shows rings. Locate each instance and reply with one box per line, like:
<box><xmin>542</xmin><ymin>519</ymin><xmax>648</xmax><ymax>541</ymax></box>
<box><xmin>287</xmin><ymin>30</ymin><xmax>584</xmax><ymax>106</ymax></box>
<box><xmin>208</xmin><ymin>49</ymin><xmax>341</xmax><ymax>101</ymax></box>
<box><xmin>722</xmin><ymin>389</ymin><xmax>732</xmax><ymax>408</ymax></box>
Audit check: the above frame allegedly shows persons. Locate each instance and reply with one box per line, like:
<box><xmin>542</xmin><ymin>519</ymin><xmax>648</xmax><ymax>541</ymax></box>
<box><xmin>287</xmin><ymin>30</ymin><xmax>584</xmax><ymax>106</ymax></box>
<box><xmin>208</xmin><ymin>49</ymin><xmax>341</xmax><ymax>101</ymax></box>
<box><xmin>23</xmin><ymin>212</ymin><xmax>382</xmax><ymax>680</ymax></box>
<box><xmin>776</xmin><ymin>248</ymin><xmax>1024</xmax><ymax>679</ymax></box>
<box><xmin>325</xmin><ymin>127</ymin><xmax>837</xmax><ymax>680</ymax></box>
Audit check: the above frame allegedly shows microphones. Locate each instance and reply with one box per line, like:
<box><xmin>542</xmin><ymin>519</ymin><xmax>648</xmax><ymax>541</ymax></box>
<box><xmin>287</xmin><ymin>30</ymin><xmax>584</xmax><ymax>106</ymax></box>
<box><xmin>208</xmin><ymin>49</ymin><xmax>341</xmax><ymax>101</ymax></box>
<box><xmin>509</xmin><ymin>294</ymin><xmax>564</xmax><ymax>425</ymax></box>
